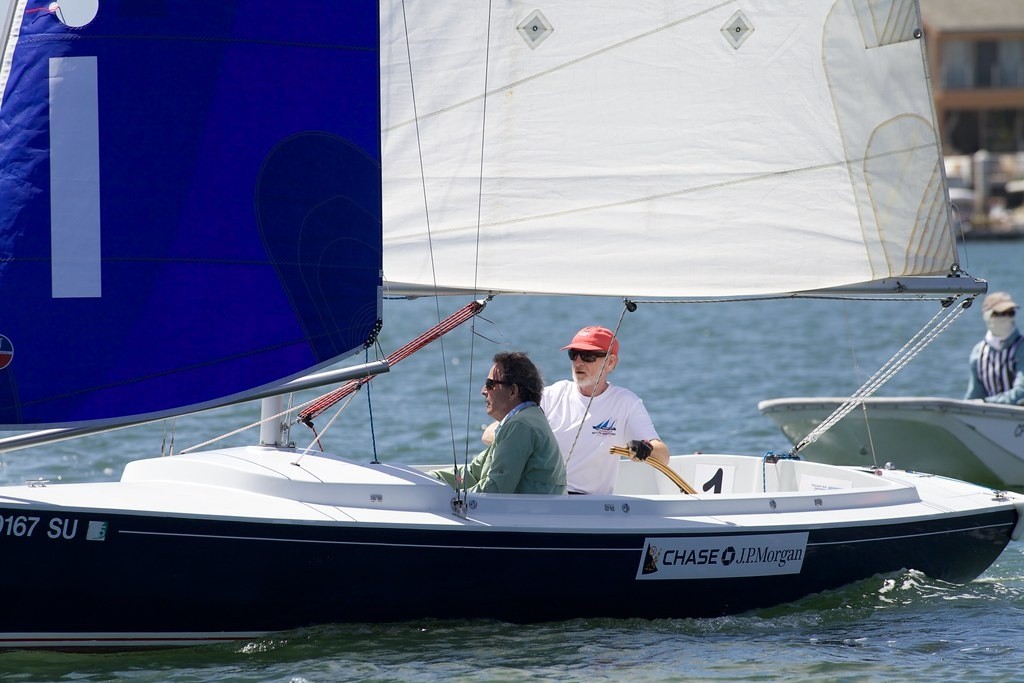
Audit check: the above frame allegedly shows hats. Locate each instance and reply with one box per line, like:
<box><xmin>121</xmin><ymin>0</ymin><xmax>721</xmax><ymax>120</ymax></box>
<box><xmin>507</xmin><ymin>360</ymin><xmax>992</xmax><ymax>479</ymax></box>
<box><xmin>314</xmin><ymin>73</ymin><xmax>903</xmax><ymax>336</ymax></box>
<box><xmin>982</xmin><ymin>292</ymin><xmax>1019</xmax><ymax>314</ymax></box>
<box><xmin>560</xmin><ymin>326</ymin><xmax>619</xmax><ymax>355</ymax></box>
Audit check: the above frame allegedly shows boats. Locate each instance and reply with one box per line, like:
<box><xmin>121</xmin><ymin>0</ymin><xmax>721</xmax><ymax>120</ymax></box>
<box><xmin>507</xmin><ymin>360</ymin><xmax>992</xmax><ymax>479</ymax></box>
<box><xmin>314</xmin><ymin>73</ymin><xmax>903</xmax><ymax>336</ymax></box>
<box><xmin>746</xmin><ymin>395</ymin><xmax>1023</xmax><ymax>500</ymax></box>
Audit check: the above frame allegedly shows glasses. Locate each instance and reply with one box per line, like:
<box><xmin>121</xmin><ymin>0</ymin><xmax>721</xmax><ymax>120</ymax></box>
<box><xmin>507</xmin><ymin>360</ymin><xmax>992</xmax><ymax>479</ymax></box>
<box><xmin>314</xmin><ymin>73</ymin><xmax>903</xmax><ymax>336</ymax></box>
<box><xmin>568</xmin><ymin>349</ymin><xmax>612</xmax><ymax>362</ymax></box>
<box><xmin>993</xmin><ymin>311</ymin><xmax>1015</xmax><ymax>317</ymax></box>
<box><xmin>486</xmin><ymin>378</ymin><xmax>520</xmax><ymax>393</ymax></box>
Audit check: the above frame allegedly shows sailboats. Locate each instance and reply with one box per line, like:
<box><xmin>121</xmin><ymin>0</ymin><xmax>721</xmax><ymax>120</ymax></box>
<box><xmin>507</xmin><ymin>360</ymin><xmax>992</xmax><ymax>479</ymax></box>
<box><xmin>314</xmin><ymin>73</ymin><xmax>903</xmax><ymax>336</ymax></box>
<box><xmin>2</xmin><ymin>0</ymin><xmax>1024</xmax><ymax>644</ymax></box>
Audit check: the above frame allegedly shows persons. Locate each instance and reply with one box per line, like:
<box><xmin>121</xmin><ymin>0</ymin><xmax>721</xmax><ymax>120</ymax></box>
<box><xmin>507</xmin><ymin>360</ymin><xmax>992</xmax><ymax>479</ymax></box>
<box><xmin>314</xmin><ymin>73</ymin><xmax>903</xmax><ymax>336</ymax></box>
<box><xmin>482</xmin><ymin>325</ymin><xmax>670</xmax><ymax>495</ymax></box>
<box><xmin>963</xmin><ymin>291</ymin><xmax>1024</xmax><ymax>409</ymax></box>
<box><xmin>423</xmin><ymin>351</ymin><xmax>567</xmax><ymax>495</ymax></box>
